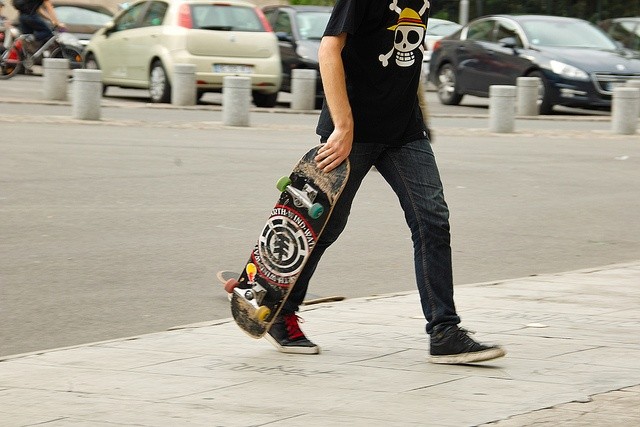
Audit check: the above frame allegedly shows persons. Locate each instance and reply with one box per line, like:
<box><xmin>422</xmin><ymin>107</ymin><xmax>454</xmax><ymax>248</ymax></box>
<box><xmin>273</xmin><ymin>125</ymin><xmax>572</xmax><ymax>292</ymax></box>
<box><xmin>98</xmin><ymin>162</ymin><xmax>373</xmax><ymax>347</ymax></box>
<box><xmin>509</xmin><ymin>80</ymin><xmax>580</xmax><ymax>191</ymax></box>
<box><xmin>12</xmin><ymin>0</ymin><xmax>65</xmax><ymax>51</ymax></box>
<box><xmin>263</xmin><ymin>0</ymin><xmax>509</xmax><ymax>365</ymax></box>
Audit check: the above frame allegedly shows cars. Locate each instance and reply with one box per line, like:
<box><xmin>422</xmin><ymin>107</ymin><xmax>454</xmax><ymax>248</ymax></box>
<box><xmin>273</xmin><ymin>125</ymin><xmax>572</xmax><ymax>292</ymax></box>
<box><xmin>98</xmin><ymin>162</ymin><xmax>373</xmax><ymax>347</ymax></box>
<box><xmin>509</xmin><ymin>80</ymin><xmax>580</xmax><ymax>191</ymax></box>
<box><xmin>427</xmin><ymin>13</ymin><xmax>638</xmax><ymax>112</ymax></box>
<box><xmin>264</xmin><ymin>4</ymin><xmax>336</xmax><ymax>107</ymax></box>
<box><xmin>38</xmin><ymin>4</ymin><xmax>116</xmax><ymax>66</ymax></box>
<box><xmin>421</xmin><ymin>18</ymin><xmax>465</xmax><ymax>80</ymax></box>
<box><xmin>599</xmin><ymin>18</ymin><xmax>638</xmax><ymax>58</ymax></box>
<box><xmin>81</xmin><ymin>1</ymin><xmax>283</xmax><ymax>106</ymax></box>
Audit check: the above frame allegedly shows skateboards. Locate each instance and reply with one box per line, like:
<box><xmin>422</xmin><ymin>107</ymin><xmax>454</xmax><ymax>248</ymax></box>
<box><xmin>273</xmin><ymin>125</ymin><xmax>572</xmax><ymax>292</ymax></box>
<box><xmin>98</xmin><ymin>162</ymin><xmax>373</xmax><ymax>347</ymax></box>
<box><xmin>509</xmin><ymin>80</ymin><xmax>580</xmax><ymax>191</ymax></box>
<box><xmin>225</xmin><ymin>143</ymin><xmax>351</xmax><ymax>340</ymax></box>
<box><xmin>216</xmin><ymin>271</ymin><xmax>346</xmax><ymax>305</ymax></box>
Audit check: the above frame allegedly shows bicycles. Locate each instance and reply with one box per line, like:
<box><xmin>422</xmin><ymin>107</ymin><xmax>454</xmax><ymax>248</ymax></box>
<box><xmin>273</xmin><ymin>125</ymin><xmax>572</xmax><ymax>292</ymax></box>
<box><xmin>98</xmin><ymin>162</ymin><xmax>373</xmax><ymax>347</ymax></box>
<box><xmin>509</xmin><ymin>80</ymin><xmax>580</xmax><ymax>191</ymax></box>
<box><xmin>0</xmin><ymin>22</ymin><xmax>80</xmax><ymax>79</ymax></box>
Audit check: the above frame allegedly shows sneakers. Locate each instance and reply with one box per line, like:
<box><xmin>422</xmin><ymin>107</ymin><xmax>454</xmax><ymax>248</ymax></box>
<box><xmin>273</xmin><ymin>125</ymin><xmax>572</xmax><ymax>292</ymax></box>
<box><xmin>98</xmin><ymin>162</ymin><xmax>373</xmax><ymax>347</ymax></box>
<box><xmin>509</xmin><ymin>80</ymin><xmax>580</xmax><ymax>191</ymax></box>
<box><xmin>267</xmin><ymin>309</ymin><xmax>320</xmax><ymax>355</ymax></box>
<box><xmin>428</xmin><ymin>323</ymin><xmax>505</xmax><ymax>365</ymax></box>
